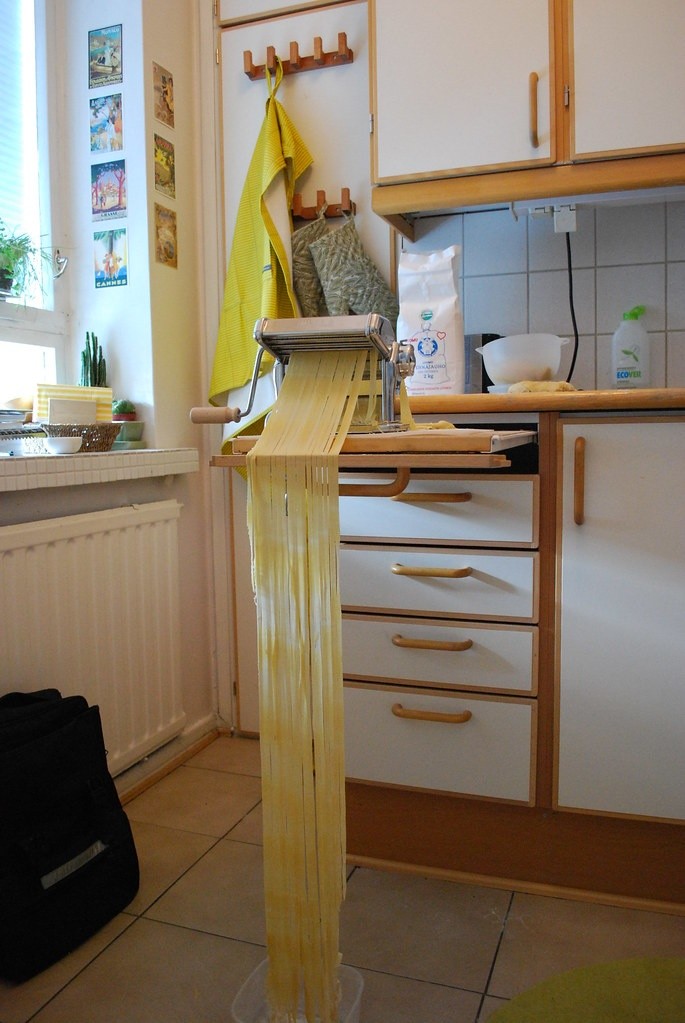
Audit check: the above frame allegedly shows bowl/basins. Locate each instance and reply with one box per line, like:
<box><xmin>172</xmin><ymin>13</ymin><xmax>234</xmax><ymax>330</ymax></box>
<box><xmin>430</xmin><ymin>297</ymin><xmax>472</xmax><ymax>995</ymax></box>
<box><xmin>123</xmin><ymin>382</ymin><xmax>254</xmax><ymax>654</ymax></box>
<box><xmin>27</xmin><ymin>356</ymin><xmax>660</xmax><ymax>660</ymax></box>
<box><xmin>112</xmin><ymin>421</ymin><xmax>145</xmax><ymax>440</ymax></box>
<box><xmin>475</xmin><ymin>333</ymin><xmax>570</xmax><ymax>386</ymax></box>
<box><xmin>42</xmin><ymin>437</ymin><xmax>82</xmax><ymax>454</ymax></box>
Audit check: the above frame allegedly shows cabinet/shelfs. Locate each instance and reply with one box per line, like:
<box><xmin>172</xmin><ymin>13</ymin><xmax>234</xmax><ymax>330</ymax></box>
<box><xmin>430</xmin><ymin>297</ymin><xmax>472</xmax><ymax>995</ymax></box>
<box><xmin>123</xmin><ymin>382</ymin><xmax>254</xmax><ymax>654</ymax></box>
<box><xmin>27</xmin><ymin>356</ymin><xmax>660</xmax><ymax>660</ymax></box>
<box><xmin>547</xmin><ymin>411</ymin><xmax>684</xmax><ymax>826</ymax></box>
<box><xmin>367</xmin><ymin>0</ymin><xmax>684</xmax><ymax>244</ymax></box>
<box><xmin>208</xmin><ymin>413</ymin><xmax>547</xmax><ymax>811</ymax></box>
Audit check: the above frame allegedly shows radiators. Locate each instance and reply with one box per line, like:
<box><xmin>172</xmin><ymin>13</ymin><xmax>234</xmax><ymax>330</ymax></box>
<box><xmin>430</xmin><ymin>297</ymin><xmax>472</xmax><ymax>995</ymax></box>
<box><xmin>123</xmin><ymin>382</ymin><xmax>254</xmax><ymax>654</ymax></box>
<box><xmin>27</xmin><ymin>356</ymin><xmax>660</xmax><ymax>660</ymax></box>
<box><xmin>0</xmin><ymin>498</ymin><xmax>185</xmax><ymax>778</ymax></box>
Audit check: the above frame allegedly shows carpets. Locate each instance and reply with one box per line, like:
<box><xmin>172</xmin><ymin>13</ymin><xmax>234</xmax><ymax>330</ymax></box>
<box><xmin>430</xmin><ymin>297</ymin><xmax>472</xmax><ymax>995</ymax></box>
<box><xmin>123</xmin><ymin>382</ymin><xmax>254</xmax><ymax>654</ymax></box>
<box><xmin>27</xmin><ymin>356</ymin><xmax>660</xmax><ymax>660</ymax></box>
<box><xmin>486</xmin><ymin>956</ymin><xmax>685</xmax><ymax>1022</ymax></box>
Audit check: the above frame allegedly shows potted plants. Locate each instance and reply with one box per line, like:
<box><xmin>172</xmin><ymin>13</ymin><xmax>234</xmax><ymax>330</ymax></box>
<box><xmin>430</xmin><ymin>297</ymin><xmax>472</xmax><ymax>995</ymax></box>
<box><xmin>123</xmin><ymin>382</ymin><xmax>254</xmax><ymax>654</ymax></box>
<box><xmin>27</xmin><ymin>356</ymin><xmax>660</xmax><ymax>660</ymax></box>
<box><xmin>111</xmin><ymin>399</ymin><xmax>136</xmax><ymax>421</ymax></box>
<box><xmin>78</xmin><ymin>331</ymin><xmax>144</xmax><ymax>441</ymax></box>
<box><xmin>0</xmin><ymin>216</ymin><xmax>76</xmax><ymax>309</ymax></box>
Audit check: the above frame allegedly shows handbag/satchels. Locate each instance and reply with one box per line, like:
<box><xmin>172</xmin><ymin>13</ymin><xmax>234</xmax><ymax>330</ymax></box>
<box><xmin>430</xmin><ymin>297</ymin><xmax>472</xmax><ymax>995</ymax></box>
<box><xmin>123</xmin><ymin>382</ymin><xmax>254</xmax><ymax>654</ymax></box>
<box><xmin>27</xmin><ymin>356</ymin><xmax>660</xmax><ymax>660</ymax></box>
<box><xmin>0</xmin><ymin>687</ymin><xmax>141</xmax><ymax>986</ymax></box>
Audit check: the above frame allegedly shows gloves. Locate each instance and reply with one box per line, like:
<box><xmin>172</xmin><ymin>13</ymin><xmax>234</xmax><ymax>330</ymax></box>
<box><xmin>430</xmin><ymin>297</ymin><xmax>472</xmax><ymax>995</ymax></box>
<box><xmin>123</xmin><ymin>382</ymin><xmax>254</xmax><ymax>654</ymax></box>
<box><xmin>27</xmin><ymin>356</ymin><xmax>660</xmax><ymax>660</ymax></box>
<box><xmin>288</xmin><ymin>215</ymin><xmax>329</xmax><ymax>317</ymax></box>
<box><xmin>308</xmin><ymin>217</ymin><xmax>399</xmax><ymax>330</ymax></box>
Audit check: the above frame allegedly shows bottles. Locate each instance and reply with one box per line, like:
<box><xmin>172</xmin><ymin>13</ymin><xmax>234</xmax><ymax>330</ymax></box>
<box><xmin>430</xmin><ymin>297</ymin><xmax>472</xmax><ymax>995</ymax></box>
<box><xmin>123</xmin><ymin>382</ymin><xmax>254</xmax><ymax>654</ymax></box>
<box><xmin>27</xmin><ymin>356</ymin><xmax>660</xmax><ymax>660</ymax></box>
<box><xmin>610</xmin><ymin>304</ymin><xmax>650</xmax><ymax>389</ymax></box>
